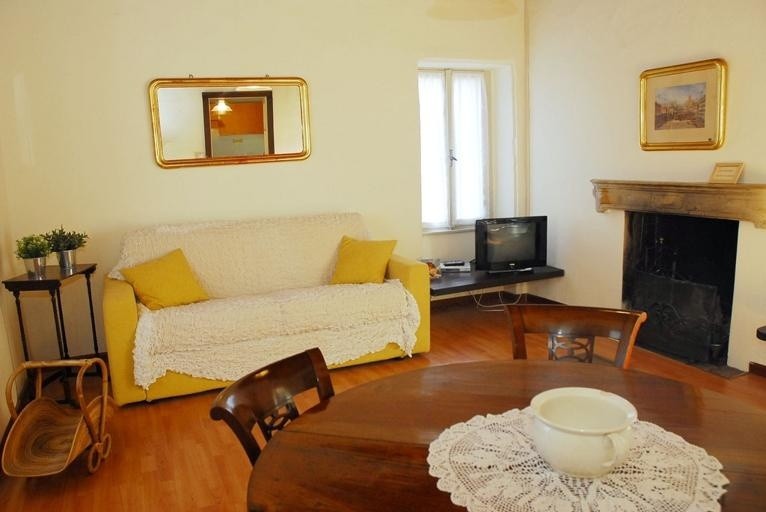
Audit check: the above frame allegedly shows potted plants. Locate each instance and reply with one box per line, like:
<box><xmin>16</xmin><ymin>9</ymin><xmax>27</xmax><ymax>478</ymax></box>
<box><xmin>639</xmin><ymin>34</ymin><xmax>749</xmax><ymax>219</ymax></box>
<box><xmin>16</xmin><ymin>234</ymin><xmax>52</xmax><ymax>279</ymax></box>
<box><xmin>46</xmin><ymin>224</ymin><xmax>89</xmax><ymax>268</ymax></box>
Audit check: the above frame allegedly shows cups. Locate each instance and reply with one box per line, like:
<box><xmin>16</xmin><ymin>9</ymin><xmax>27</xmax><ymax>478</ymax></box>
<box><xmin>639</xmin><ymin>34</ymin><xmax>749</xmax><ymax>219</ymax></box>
<box><xmin>530</xmin><ymin>386</ymin><xmax>640</xmax><ymax>480</ymax></box>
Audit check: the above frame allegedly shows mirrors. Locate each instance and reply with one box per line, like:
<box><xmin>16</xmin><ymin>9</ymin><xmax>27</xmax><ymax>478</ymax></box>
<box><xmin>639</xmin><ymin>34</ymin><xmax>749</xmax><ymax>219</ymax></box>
<box><xmin>147</xmin><ymin>74</ymin><xmax>312</xmax><ymax>170</ymax></box>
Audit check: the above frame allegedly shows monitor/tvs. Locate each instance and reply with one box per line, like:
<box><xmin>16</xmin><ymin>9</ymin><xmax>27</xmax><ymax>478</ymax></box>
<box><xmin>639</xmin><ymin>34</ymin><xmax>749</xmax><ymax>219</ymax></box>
<box><xmin>476</xmin><ymin>216</ymin><xmax>547</xmax><ymax>273</ymax></box>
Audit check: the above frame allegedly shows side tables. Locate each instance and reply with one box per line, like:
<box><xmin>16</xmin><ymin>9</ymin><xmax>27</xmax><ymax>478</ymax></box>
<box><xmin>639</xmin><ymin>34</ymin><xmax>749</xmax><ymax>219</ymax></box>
<box><xmin>2</xmin><ymin>263</ymin><xmax>109</xmax><ymax>409</ymax></box>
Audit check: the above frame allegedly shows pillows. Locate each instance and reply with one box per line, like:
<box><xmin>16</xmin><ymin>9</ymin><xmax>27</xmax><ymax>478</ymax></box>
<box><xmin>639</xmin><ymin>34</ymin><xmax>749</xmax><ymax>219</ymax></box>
<box><xmin>119</xmin><ymin>248</ymin><xmax>211</xmax><ymax>311</ymax></box>
<box><xmin>328</xmin><ymin>235</ymin><xmax>397</xmax><ymax>285</ymax></box>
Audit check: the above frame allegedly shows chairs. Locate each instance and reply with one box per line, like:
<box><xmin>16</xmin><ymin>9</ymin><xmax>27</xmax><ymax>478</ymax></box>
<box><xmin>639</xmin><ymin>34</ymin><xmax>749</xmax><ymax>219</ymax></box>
<box><xmin>209</xmin><ymin>347</ymin><xmax>335</xmax><ymax>468</ymax></box>
<box><xmin>505</xmin><ymin>304</ymin><xmax>647</xmax><ymax>369</ymax></box>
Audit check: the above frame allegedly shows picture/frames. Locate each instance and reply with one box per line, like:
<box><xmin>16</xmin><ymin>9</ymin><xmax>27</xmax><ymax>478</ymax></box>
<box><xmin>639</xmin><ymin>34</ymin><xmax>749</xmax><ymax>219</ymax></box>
<box><xmin>639</xmin><ymin>58</ymin><xmax>728</xmax><ymax>151</ymax></box>
<box><xmin>709</xmin><ymin>161</ymin><xmax>744</xmax><ymax>185</ymax></box>
<box><xmin>201</xmin><ymin>90</ymin><xmax>274</xmax><ymax>158</ymax></box>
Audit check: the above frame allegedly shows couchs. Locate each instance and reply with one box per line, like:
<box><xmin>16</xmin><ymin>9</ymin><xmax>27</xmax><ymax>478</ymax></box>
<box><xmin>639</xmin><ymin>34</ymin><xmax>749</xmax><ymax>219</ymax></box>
<box><xmin>102</xmin><ymin>213</ymin><xmax>430</xmax><ymax>408</ymax></box>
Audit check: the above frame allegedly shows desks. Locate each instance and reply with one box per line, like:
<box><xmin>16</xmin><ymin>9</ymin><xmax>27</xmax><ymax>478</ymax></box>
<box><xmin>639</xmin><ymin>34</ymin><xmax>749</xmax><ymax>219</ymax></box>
<box><xmin>430</xmin><ymin>262</ymin><xmax>564</xmax><ymax>296</ymax></box>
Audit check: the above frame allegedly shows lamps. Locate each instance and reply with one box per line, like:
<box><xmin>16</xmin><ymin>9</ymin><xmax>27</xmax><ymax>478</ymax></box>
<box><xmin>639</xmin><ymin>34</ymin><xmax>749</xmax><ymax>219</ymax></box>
<box><xmin>211</xmin><ymin>100</ymin><xmax>233</xmax><ymax>115</ymax></box>
